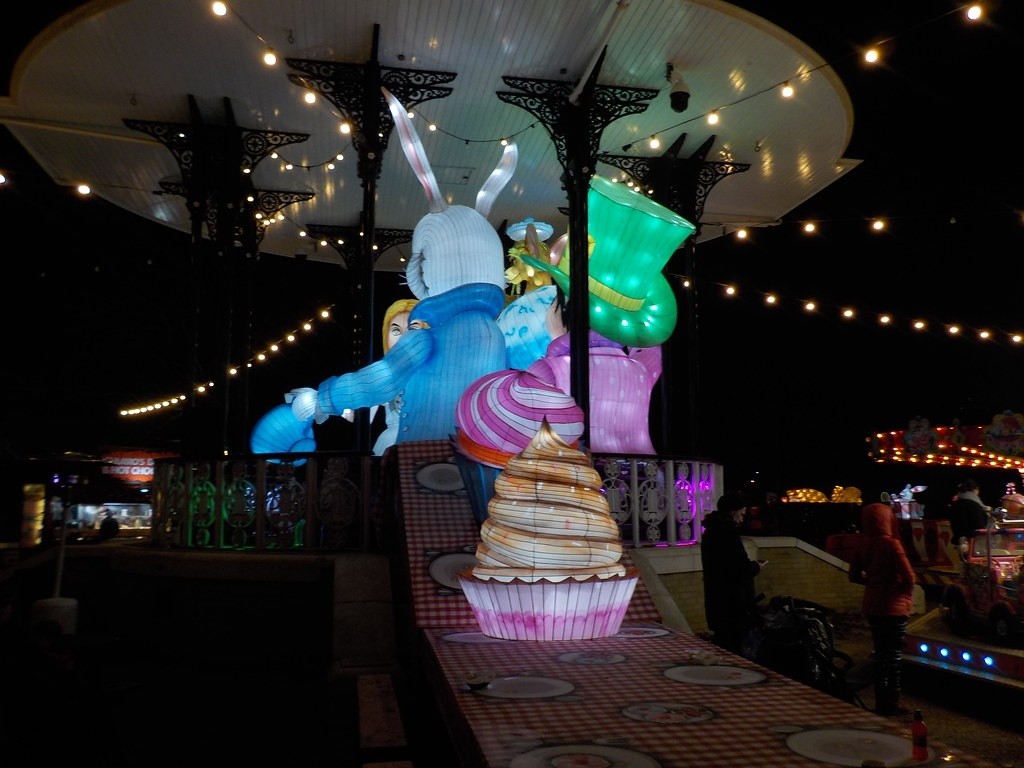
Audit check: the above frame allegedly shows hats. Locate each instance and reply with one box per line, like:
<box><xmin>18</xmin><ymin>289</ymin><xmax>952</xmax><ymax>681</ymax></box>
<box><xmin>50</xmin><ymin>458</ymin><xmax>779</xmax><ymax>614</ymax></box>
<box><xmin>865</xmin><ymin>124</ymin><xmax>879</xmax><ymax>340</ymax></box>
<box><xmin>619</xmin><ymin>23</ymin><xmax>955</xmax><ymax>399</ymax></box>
<box><xmin>718</xmin><ymin>494</ymin><xmax>748</xmax><ymax>514</ymax></box>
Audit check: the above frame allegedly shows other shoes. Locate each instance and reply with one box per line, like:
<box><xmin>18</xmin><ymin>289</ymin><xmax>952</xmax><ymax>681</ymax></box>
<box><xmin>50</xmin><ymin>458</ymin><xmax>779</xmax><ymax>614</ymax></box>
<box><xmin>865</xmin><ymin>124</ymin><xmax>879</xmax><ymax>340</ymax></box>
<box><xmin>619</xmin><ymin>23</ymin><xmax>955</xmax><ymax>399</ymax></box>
<box><xmin>875</xmin><ymin>701</ymin><xmax>913</xmax><ymax>716</ymax></box>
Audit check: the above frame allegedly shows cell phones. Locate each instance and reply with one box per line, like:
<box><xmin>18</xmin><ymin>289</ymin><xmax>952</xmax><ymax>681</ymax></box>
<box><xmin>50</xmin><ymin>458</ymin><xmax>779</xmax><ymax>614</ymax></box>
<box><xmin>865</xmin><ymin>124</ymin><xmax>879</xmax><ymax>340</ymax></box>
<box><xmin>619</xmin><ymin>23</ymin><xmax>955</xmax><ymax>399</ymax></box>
<box><xmin>759</xmin><ymin>561</ymin><xmax>768</xmax><ymax>565</ymax></box>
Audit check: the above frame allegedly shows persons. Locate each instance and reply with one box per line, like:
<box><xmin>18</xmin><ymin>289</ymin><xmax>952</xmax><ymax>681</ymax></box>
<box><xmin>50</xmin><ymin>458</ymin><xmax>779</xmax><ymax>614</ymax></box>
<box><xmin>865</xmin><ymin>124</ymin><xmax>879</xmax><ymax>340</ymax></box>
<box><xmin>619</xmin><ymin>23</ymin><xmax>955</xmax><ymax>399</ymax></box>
<box><xmin>100</xmin><ymin>511</ymin><xmax>120</xmax><ymax>539</ymax></box>
<box><xmin>949</xmin><ymin>478</ymin><xmax>993</xmax><ymax>546</ymax></box>
<box><xmin>699</xmin><ymin>488</ymin><xmax>766</xmax><ymax>656</ymax></box>
<box><xmin>365</xmin><ymin>300</ymin><xmax>425</xmax><ymax>457</ymax></box>
<box><xmin>848</xmin><ymin>503</ymin><xmax>917</xmax><ymax>714</ymax></box>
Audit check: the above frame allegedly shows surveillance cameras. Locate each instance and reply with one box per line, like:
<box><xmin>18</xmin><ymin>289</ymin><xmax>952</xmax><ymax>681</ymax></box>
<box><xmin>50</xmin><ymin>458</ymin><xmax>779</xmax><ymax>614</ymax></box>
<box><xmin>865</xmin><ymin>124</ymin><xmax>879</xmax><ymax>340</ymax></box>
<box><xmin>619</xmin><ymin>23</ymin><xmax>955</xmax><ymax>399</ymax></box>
<box><xmin>669</xmin><ymin>81</ymin><xmax>691</xmax><ymax>114</ymax></box>
